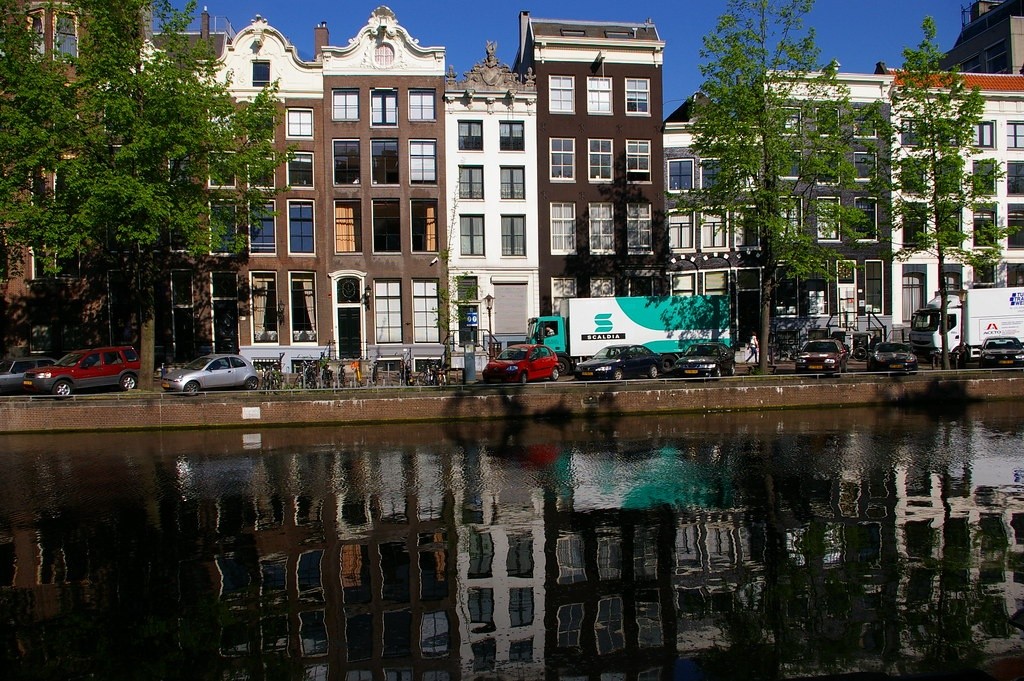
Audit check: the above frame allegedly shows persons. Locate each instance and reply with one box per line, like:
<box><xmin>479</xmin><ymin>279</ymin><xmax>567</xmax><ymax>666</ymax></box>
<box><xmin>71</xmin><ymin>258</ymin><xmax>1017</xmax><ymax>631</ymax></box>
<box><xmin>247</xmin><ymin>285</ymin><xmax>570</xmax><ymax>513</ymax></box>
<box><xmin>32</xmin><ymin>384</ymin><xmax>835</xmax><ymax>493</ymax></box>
<box><xmin>745</xmin><ymin>332</ymin><xmax>759</xmax><ymax>363</ymax></box>
<box><xmin>546</xmin><ymin>325</ymin><xmax>555</xmax><ymax>336</ymax></box>
<box><xmin>158</xmin><ymin>361</ymin><xmax>444</xmax><ymax>395</ymax></box>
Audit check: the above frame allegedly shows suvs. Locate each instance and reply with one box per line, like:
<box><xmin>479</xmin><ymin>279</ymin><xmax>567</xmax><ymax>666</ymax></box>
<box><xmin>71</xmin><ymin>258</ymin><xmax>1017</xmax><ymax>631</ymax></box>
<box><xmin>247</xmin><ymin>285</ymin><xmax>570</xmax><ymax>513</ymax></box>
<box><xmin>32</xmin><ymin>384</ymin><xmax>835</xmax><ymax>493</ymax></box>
<box><xmin>22</xmin><ymin>344</ymin><xmax>141</xmax><ymax>401</ymax></box>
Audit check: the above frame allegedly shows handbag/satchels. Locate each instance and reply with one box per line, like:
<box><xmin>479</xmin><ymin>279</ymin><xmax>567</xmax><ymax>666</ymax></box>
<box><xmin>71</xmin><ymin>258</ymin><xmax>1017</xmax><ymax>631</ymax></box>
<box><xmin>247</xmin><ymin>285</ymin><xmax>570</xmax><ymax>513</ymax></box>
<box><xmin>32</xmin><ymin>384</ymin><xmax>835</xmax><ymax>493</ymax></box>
<box><xmin>750</xmin><ymin>335</ymin><xmax>757</xmax><ymax>346</ymax></box>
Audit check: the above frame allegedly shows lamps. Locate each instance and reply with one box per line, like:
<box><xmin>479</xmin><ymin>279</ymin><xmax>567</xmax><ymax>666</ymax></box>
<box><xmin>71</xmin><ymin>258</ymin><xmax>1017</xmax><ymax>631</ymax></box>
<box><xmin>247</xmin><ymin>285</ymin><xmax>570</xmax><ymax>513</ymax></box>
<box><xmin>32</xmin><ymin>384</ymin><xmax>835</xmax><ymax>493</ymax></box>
<box><xmin>365</xmin><ymin>285</ymin><xmax>371</xmax><ymax>311</ymax></box>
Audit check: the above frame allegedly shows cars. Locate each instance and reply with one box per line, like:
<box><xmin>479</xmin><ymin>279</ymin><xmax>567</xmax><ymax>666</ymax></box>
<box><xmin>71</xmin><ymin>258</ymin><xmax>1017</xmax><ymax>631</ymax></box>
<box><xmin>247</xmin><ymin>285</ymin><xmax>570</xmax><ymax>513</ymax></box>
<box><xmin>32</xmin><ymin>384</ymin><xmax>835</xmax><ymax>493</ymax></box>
<box><xmin>573</xmin><ymin>344</ymin><xmax>664</xmax><ymax>381</ymax></box>
<box><xmin>867</xmin><ymin>340</ymin><xmax>919</xmax><ymax>376</ymax></box>
<box><xmin>482</xmin><ymin>344</ymin><xmax>561</xmax><ymax>386</ymax></box>
<box><xmin>674</xmin><ymin>342</ymin><xmax>737</xmax><ymax>381</ymax></box>
<box><xmin>795</xmin><ymin>339</ymin><xmax>849</xmax><ymax>378</ymax></box>
<box><xmin>0</xmin><ymin>355</ymin><xmax>58</xmax><ymax>393</ymax></box>
<box><xmin>978</xmin><ymin>335</ymin><xmax>1024</xmax><ymax>369</ymax></box>
<box><xmin>161</xmin><ymin>353</ymin><xmax>260</xmax><ymax>397</ymax></box>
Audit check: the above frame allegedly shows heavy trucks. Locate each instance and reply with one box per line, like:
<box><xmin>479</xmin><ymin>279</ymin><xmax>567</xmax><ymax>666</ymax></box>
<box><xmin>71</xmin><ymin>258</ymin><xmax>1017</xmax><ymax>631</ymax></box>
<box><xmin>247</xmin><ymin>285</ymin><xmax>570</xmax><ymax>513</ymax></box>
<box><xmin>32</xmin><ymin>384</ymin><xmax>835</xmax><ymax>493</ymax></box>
<box><xmin>525</xmin><ymin>293</ymin><xmax>733</xmax><ymax>376</ymax></box>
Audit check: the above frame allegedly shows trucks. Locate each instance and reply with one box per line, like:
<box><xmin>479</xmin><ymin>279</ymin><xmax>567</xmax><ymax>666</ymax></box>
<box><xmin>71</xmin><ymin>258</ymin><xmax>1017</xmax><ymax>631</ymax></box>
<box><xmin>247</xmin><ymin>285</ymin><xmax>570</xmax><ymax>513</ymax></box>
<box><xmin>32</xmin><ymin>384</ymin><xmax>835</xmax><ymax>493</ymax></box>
<box><xmin>908</xmin><ymin>286</ymin><xmax>1024</xmax><ymax>366</ymax></box>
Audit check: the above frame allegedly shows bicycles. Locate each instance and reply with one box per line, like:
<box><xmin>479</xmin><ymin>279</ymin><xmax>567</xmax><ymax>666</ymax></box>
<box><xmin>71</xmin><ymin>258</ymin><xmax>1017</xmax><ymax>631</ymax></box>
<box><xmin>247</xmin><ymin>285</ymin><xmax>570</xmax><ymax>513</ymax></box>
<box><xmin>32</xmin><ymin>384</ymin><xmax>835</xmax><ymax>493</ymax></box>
<box><xmin>262</xmin><ymin>363</ymin><xmax>452</xmax><ymax>396</ymax></box>
